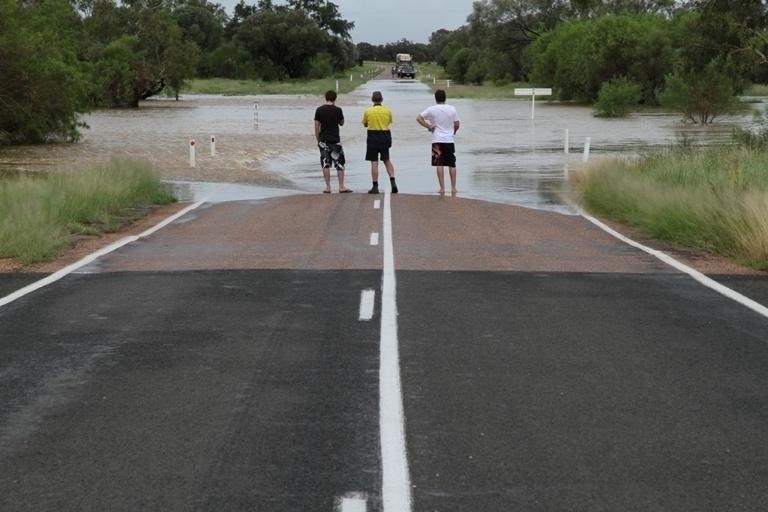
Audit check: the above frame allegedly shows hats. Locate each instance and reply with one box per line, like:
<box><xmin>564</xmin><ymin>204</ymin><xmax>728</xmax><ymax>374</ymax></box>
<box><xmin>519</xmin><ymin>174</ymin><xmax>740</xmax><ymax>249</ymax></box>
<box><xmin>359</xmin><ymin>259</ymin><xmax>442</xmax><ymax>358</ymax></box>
<box><xmin>372</xmin><ymin>90</ymin><xmax>383</xmax><ymax>105</ymax></box>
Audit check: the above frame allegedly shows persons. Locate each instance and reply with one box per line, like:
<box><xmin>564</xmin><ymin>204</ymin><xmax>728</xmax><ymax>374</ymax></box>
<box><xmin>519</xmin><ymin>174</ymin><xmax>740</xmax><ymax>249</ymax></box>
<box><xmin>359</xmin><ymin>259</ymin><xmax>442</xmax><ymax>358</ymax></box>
<box><xmin>314</xmin><ymin>90</ymin><xmax>353</xmax><ymax>193</ymax></box>
<box><xmin>362</xmin><ymin>91</ymin><xmax>398</xmax><ymax>194</ymax></box>
<box><xmin>416</xmin><ymin>90</ymin><xmax>460</xmax><ymax>193</ymax></box>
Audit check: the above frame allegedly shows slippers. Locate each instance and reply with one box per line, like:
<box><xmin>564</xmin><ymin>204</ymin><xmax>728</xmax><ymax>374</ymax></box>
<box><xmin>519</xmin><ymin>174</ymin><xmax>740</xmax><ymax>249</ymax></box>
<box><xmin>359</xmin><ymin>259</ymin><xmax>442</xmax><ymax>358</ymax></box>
<box><xmin>340</xmin><ymin>188</ymin><xmax>354</xmax><ymax>193</ymax></box>
<box><xmin>322</xmin><ymin>190</ymin><xmax>331</xmax><ymax>193</ymax></box>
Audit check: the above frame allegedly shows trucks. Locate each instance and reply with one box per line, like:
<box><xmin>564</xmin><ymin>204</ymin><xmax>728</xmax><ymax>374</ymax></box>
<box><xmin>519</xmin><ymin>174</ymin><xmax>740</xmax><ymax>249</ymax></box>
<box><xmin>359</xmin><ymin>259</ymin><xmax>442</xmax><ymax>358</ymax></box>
<box><xmin>396</xmin><ymin>53</ymin><xmax>414</xmax><ymax>67</ymax></box>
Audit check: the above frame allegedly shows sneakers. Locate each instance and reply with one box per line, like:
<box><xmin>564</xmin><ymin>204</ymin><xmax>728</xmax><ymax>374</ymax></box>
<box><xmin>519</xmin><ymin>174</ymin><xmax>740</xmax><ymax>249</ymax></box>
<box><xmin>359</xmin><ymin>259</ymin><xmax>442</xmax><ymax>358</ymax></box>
<box><xmin>367</xmin><ymin>186</ymin><xmax>380</xmax><ymax>194</ymax></box>
<box><xmin>391</xmin><ymin>184</ymin><xmax>399</xmax><ymax>193</ymax></box>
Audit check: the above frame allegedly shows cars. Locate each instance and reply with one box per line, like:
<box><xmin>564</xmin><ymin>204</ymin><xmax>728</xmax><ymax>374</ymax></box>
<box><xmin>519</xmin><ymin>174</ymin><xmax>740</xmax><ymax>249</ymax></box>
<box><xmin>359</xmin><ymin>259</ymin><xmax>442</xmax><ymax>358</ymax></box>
<box><xmin>391</xmin><ymin>64</ymin><xmax>416</xmax><ymax>80</ymax></box>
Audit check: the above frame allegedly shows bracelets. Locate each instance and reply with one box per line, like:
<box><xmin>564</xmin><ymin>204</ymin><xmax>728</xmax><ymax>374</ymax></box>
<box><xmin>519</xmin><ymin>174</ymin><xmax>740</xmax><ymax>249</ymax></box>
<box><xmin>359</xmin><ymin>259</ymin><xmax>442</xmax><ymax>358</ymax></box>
<box><xmin>429</xmin><ymin>127</ymin><xmax>432</xmax><ymax>131</ymax></box>
